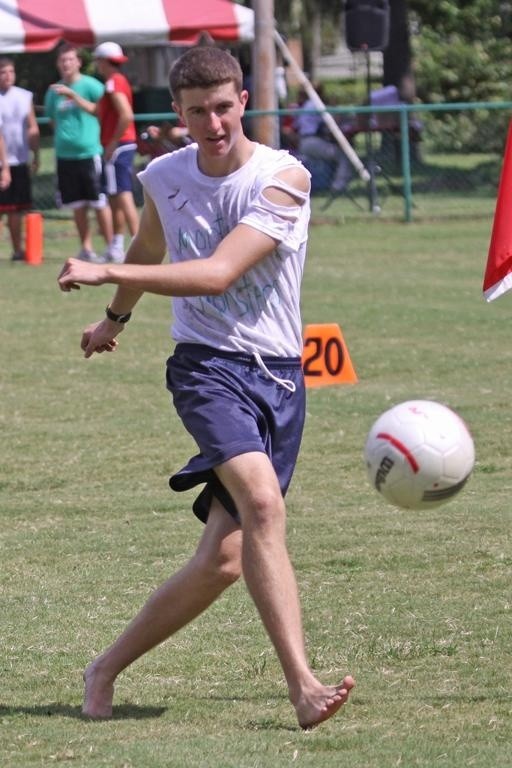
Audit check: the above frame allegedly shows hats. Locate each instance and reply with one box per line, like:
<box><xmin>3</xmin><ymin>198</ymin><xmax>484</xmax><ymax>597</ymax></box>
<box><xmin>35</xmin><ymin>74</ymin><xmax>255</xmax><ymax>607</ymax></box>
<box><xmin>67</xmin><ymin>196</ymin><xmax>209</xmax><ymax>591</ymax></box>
<box><xmin>91</xmin><ymin>41</ymin><xmax>129</xmax><ymax>63</ymax></box>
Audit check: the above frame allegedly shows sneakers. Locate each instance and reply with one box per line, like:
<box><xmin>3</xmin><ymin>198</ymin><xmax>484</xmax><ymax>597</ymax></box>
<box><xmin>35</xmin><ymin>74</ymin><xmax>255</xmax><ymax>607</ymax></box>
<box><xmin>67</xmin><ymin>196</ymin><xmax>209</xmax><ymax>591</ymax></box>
<box><xmin>11</xmin><ymin>252</ymin><xmax>26</xmax><ymax>262</ymax></box>
<box><xmin>77</xmin><ymin>250</ymin><xmax>104</xmax><ymax>263</ymax></box>
<box><xmin>107</xmin><ymin>246</ymin><xmax>125</xmax><ymax>264</ymax></box>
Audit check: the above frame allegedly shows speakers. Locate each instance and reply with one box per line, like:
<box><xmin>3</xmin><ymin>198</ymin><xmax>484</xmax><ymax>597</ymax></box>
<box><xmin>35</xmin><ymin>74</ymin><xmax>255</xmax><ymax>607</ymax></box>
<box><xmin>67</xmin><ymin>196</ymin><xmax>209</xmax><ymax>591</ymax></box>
<box><xmin>345</xmin><ymin>0</ymin><xmax>392</xmax><ymax>53</ymax></box>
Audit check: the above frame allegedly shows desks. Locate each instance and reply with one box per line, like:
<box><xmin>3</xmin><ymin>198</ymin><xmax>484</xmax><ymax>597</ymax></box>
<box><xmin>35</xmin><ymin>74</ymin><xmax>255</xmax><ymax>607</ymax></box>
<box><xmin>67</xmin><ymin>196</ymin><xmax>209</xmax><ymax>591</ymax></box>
<box><xmin>320</xmin><ymin>124</ymin><xmax>419</xmax><ymax>212</ymax></box>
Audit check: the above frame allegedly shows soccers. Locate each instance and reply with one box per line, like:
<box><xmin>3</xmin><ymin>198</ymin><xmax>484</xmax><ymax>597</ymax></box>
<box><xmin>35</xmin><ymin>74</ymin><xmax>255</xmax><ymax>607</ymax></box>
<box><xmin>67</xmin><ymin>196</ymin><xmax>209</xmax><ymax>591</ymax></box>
<box><xmin>363</xmin><ymin>399</ymin><xmax>476</xmax><ymax>512</ymax></box>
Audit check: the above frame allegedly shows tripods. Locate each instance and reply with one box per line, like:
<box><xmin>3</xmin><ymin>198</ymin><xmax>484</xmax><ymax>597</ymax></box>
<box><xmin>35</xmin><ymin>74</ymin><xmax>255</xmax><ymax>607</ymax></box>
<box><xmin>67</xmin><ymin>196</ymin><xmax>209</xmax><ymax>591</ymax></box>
<box><xmin>317</xmin><ymin>53</ymin><xmax>417</xmax><ymax>213</ymax></box>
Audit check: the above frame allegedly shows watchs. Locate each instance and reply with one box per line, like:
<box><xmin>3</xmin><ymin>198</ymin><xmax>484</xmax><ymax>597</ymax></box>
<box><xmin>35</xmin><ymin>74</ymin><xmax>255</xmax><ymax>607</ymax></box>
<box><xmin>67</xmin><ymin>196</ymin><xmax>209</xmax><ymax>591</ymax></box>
<box><xmin>105</xmin><ymin>304</ymin><xmax>132</xmax><ymax>323</ymax></box>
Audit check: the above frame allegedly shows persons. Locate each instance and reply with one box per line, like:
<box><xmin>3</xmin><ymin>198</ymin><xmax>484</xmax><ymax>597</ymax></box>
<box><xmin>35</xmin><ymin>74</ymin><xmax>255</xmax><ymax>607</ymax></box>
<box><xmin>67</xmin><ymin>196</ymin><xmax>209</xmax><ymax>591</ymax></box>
<box><xmin>135</xmin><ymin>82</ymin><xmax>359</xmax><ymax>197</ymax></box>
<box><xmin>49</xmin><ymin>36</ymin><xmax>144</xmax><ymax>268</ymax></box>
<box><xmin>0</xmin><ymin>52</ymin><xmax>43</xmax><ymax>265</ymax></box>
<box><xmin>55</xmin><ymin>42</ymin><xmax>358</xmax><ymax>732</ymax></box>
<box><xmin>41</xmin><ymin>41</ymin><xmax>118</xmax><ymax>267</ymax></box>
